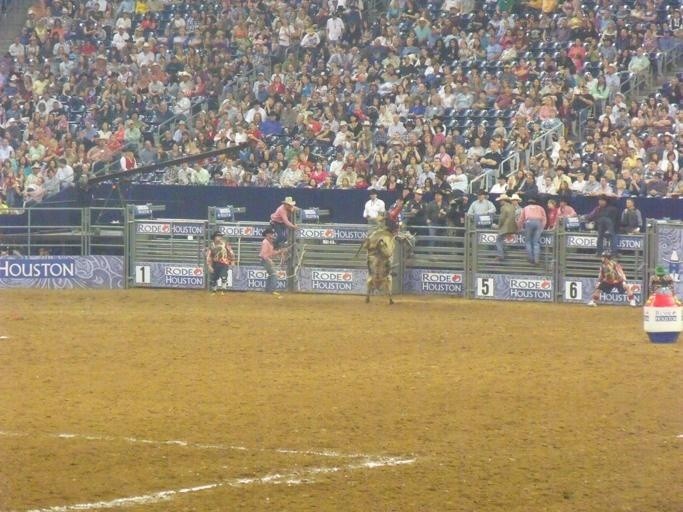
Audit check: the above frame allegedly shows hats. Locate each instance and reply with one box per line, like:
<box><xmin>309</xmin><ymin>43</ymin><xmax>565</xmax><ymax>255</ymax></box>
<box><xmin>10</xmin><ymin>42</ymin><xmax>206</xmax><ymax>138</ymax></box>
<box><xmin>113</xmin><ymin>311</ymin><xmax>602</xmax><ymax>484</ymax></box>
<box><xmin>223</xmin><ymin>99</ymin><xmax>277</xmax><ymax>116</ymax></box>
<box><xmin>656</xmin><ymin>266</ymin><xmax>664</xmax><ymax>275</ymax></box>
<box><xmin>182</xmin><ymin>72</ymin><xmax>193</xmax><ymax>79</ymax></box>
<box><xmin>262</xmin><ymin>229</ymin><xmax>274</xmax><ymax>236</ymax></box>
<box><xmin>614</xmin><ymin>92</ymin><xmax>625</xmax><ymax>99</ymax></box>
<box><xmin>462</xmin><ymin>82</ymin><xmax>469</xmax><ymax>87</ymax></box>
<box><xmin>476</xmin><ymin>189</ymin><xmax>538</xmax><ymax>204</ymax></box>
<box><xmin>281</xmin><ymin>197</ymin><xmax>296</xmax><ymax>206</ymax></box>
<box><xmin>608</xmin><ymin>145</ymin><xmax>617</xmax><ymax>152</ymax></box>
<box><xmin>79</xmin><ymin>54</ymin><xmax>119</xmax><ymax>79</ymax></box>
<box><xmin>413</xmin><ymin>174</ymin><xmax>444</xmax><ymax>195</ymax></box>
<box><xmin>340</xmin><ymin>120</ymin><xmax>371</xmax><ymax>126</ymax></box>
<box><xmin>118</xmin><ymin>25</ymin><xmax>160</xmax><ymax>66</ymax></box>
<box><xmin>431</xmin><ymin>115</ymin><xmax>444</xmax><ymax>132</ymax></box>
<box><xmin>376</xmin><ymin>140</ymin><xmax>402</xmax><ymax>149</ymax></box>
<box><xmin>664</xmin><ymin>132</ymin><xmax>673</xmax><ymax>139</ymax></box>
<box><xmin>211</xmin><ymin>231</ymin><xmax>223</xmax><ymax>240</ymax></box>
<box><xmin>258</xmin><ymin>159</ymin><xmax>268</xmax><ymax>167</ymax></box>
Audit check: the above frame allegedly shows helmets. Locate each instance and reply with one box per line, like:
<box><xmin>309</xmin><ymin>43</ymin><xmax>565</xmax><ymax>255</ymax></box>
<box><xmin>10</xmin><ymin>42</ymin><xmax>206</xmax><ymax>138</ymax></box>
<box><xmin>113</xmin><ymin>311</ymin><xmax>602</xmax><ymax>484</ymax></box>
<box><xmin>602</xmin><ymin>251</ymin><xmax>612</xmax><ymax>257</ymax></box>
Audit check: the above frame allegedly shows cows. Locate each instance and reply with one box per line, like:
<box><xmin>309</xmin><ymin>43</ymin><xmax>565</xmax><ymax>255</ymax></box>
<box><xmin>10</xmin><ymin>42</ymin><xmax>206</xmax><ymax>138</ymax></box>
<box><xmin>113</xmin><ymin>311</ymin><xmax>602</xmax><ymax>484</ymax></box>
<box><xmin>362</xmin><ymin>217</ymin><xmax>400</xmax><ymax>305</ymax></box>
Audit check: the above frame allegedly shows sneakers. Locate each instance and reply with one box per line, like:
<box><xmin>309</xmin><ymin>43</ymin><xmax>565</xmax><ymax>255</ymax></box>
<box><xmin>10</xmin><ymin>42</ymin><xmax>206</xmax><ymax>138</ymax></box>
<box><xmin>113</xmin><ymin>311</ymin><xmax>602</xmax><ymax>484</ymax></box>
<box><xmin>588</xmin><ymin>301</ymin><xmax>597</xmax><ymax>306</ymax></box>
<box><xmin>272</xmin><ymin>292</ymin><xmax>280</xmax><ymax>299</ymax></box>
<box><xmin>630</xmin><ymin>299</ymin><xmax>636</xmax><ymax>306</ymax></box>
<box><xmin>212</xmin><ymin>291</ymin><xmax>224</xmax><ymax>296</ymax></box>
